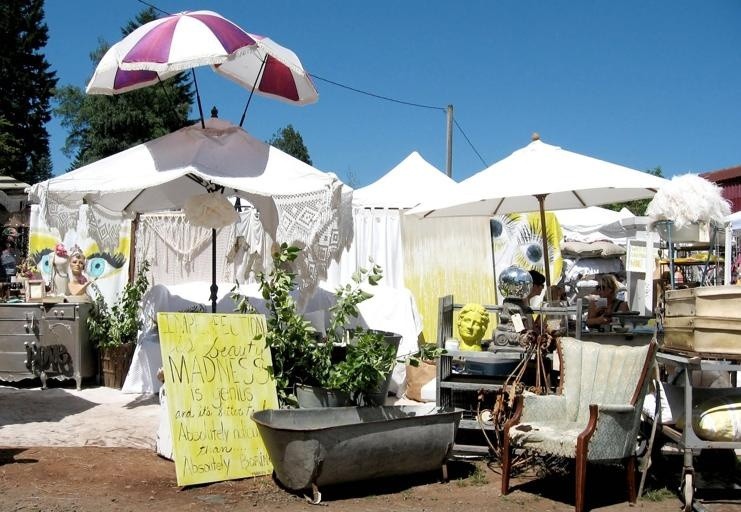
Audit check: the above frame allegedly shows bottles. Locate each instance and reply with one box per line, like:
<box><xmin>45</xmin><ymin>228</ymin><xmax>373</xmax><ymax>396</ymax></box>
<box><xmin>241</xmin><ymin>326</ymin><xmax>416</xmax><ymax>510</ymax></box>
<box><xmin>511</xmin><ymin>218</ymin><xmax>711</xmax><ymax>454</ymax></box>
<box><xmin>675</xmin><ymin>266</ymin><xmax>683</xmax><ymax>285</ymax></box>
<box><xmin>539</xmin><ymin>301</ymin><xmax>569</xmax><ymax>338</ymax></box>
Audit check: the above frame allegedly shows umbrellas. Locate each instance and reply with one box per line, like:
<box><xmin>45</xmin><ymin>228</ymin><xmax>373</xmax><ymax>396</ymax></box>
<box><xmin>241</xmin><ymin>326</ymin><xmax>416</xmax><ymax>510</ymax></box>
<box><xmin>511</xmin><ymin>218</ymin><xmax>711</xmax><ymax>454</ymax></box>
<box><xmin>82</xmin><ymin>11</ymin><xmax>322</xmax><ymax>132</ymax></box>
<box><xmin>401</xmin><ymin>136</ymin><xmax>681</xmax><ymax>306</ymax></box>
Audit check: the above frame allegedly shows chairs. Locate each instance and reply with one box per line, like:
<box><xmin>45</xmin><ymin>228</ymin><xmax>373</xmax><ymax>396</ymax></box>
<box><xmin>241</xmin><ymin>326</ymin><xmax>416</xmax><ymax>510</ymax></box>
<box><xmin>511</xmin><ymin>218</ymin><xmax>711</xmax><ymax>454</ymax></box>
<box><xmin>498</xmin><ymin>336</ymin><xmax>659</xmax><ymax>511</ymax></box>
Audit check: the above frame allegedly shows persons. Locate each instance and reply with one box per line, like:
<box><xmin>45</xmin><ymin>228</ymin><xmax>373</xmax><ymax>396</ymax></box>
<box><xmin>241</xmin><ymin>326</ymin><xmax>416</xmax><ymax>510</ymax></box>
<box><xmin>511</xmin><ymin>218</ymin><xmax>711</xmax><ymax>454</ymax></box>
<box><xmin>454</xmin><ymin>301</ymin><xmax>491</xmax><ymax>355</ymax></box>
<box><xmin>587</xmin><ymin>270</ymin><xmax>632</xmax><ymax>325</ymax></box>
<box><xmin>500</xmin><ymin>264</ymin><xmax>549</xmax><ymax>335</ymax></box>
<box><xmin>66</xmin><ymin>252</ymin><xmax>92</xmax><ymax>296</ymax></box>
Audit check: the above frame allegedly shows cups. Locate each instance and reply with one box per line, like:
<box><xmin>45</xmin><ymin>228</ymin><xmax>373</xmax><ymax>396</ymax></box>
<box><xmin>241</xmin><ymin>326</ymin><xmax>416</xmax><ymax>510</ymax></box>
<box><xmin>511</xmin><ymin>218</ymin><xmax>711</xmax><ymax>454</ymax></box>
<box><xmin>444</xmin><ymin>339</ymin><xmax>459</xmax><ymax>353</ymax></box>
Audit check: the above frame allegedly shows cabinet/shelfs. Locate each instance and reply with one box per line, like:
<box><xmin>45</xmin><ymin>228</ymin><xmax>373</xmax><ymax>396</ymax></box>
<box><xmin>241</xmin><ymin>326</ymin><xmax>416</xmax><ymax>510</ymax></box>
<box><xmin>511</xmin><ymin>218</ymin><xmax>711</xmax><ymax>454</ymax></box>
<box><xmin>2</xmin><ymin>304</ymin><xmax>91</xmax><ymax>390</ymax></box>
<box><xmin>655</xmin><ymin>349</ymin><xmax>741</xmax><ymax>506</ymax></box>
<box><xmin>436</xmin><ymin>291</ymin><xmax>585</xmax><ymax>463</ymax></box>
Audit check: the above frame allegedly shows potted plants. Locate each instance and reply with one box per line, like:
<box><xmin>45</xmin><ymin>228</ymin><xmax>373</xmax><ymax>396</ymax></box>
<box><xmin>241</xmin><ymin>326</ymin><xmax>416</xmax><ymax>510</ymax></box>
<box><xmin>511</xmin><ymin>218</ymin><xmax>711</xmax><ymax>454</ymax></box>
<box><xmin>251</xmin><ymin>241</ymin><xmax>435</xmax><ymax>410</ymax></box>
<box><xmin>85</xmin><ymin>259</ymin><xmax>154</xmax><ymax>391</ymax></box>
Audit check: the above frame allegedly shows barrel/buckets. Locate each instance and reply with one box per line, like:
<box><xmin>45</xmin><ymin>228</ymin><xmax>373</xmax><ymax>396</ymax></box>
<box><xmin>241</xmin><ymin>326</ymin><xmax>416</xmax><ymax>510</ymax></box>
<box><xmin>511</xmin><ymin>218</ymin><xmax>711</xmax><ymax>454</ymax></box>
<box><xmin>295</xmin><ymin>384</ymin><xmax>345</xmax><ymax>407</ymax></box>
<box><xmin>96</xmin><ymin>341</ymin><xmax>135</xmax><ymax>388</ymax></box>
<box><xmin>350</xmin><ymin>328</ymin><xmax>403</xmax><ymax>406</ymax></box>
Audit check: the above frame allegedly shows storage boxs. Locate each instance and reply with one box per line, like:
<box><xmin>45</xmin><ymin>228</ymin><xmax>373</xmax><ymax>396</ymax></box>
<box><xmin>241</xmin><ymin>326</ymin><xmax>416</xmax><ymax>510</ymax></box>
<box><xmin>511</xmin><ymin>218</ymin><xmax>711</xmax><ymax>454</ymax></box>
<box><xmin>657</xmin><ymin>287</ymin><xmax>740</xmax><ymax>349</ymax></box>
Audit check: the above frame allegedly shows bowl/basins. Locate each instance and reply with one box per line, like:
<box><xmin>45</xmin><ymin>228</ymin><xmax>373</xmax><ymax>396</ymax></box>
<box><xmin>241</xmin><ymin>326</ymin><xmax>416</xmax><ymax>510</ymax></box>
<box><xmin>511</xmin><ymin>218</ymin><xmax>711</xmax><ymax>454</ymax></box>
<box><xmin>613</xmin><ymin>327</ymin><xmax>629</xmax><ymax>333</ymax></box>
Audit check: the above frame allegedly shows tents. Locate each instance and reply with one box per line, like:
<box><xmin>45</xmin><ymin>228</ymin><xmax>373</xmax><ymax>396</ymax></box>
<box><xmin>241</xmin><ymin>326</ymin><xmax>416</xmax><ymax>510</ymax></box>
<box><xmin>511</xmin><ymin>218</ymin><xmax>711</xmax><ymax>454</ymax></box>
<box><xmin>19</xmin><ymin>117</ymin><xmax>351</xmax><ymax>385</ymax></box>
<box><xmin>324</xmin><ymin>149</ymin><xmax>496</xmax><ymax>360</ymax></box>
